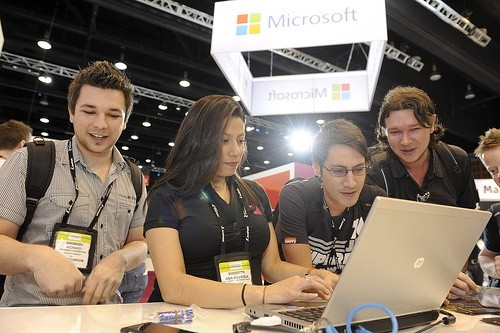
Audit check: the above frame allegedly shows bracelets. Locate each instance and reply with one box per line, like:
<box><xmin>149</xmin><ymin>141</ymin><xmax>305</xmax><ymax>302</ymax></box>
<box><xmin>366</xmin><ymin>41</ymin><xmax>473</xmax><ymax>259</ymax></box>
<box><xmin>241</xmin><ymin>284</ymin><xmax>247</xmax><ymax>307</ymax></box>
<box><xmin>262</xmin><ymin>285</ymin><xmax>267</xmax><ymax>304</ymax></box>
<box><xmin>307</xmin><ymin>267</ymin><xmax>313</xmax><ymax>275</ymax></box>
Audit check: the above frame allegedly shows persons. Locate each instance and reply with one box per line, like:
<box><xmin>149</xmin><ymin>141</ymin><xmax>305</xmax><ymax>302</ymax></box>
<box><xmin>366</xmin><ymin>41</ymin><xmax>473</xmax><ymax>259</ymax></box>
<box><xmin>117</xmin><ymin>261</ymin><xmax>148</xmax><ymax>303</ymax></box>
<box><xmin>143</xmin><ymin>95</ymin><xmax>340</xmax><ymax>307</ymax></box>
<box><xmin>0</xmin><ymin>119</ymin><xmax>33</xmax><ymax>167</ymax></box>
<box><xmin>274</xmin><ymin>86</ymin><xmax>500</xmax><ymax>306</ymax></box>
<box><xmin>0</xmin><ymin>61</ymin><xmax>148</xmax><ymax>307</ymax></box>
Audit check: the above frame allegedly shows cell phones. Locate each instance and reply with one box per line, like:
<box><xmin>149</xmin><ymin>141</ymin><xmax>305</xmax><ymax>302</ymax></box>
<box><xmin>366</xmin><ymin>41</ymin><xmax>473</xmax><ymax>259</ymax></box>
<box><xmin>120</xmin><ymin>322</ymin><xmax>198</xmax><ymax>333</ymax></box>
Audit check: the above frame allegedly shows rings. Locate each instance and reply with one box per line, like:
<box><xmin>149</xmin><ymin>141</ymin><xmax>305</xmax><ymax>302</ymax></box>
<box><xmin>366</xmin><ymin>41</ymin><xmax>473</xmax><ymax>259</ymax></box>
<box><xmin>304</xmin><ymin>273</ymin><xmax>310</xmax><ymax>281</ymax></box>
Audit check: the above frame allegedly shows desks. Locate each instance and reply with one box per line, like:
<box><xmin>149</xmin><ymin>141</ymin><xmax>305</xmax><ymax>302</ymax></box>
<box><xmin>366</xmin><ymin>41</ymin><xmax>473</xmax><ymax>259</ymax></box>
<box><xmin>0</xmin><ymin>302</ymin><xmax>500</xmax><ymax>333</ymax></box>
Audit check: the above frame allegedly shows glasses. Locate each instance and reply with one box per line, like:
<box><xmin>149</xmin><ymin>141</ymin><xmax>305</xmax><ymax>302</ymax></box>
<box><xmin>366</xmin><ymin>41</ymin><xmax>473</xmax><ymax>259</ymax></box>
<box><xmin>321</xmin><ymin>161</ymin><xmax>370</xmax><ymax>177</ymax></box>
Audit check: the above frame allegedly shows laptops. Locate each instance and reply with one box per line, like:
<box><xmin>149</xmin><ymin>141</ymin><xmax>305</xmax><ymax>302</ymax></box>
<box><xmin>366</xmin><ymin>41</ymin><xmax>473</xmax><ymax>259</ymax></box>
<box><xmin>244</xmin><ymin>196</ymin><xmax>492</xmax><ymax>333</ymax></box>
